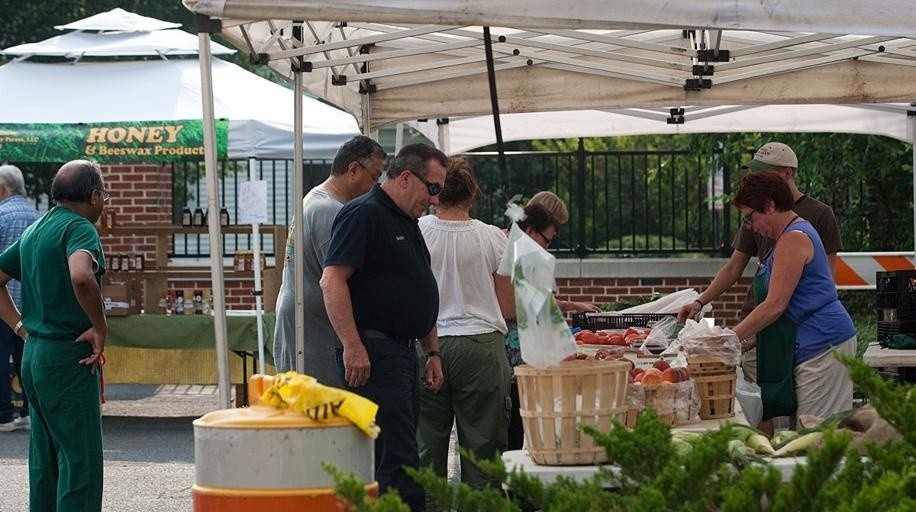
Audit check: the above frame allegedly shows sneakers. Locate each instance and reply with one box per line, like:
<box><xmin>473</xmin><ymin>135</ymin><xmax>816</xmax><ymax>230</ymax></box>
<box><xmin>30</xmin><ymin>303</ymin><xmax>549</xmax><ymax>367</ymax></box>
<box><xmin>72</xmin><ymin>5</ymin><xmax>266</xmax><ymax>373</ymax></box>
<box><xmin>0</xmin><ymin>416</ymin><xmax>32</xmax><ymax>432</ymax></box>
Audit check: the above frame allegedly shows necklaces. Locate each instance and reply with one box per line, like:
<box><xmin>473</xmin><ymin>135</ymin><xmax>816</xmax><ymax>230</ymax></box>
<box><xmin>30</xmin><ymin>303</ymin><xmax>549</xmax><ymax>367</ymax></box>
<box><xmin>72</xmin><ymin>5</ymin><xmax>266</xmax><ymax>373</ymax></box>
<box><xmin>755</xmin><ymin>215</ymin><xmax>803</xmax><ymax>268</ymax></box>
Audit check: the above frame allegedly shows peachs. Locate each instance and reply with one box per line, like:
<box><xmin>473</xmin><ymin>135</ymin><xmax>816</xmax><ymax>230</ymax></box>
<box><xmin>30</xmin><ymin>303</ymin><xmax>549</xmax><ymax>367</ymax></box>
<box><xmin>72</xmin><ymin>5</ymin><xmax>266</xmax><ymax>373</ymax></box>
<box><xmin>565</xmin><ymin>328</ymin><xmax>689</xmax><ymax>385</ymax></box>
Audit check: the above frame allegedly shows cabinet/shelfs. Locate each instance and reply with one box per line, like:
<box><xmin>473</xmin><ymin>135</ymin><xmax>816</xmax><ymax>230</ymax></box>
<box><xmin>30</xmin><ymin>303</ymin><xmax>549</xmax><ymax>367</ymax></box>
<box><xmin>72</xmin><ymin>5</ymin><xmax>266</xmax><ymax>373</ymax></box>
<box><xmin>89</xmin><ymin>225</ymin><xmax>285</xmax><ymax>318</ymax></box>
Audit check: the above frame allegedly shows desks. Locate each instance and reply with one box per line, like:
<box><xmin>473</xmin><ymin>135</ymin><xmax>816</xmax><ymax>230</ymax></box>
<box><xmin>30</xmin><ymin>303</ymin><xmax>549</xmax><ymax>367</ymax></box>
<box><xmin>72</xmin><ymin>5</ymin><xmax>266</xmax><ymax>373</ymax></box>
<box><xmin>10</xmin><ymin>313</ymin><xmax>276</xmax><ymax>413</ymax></box>
<box><xmin>862</xmin><ymin>341</ymin><xmax>916</xmax><ymax>367</ymax></box>
<box><xmin>502</xmin><ymin>325</ymin><xmax>758</xmax><ymax>486</ymax></box>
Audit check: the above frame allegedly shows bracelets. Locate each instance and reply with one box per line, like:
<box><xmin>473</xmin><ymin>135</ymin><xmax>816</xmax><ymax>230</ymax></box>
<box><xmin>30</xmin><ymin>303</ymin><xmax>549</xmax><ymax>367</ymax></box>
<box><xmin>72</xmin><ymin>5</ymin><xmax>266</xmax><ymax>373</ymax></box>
<box><xmin>13</xmin><ymin>320</ymin><xmax>23</xmax><ymax>335</ymax></box>
<box><xmin>694</xmin><ymin>299</ymin><xmax>703</xmax><ymax>308</ymax></box>
<box><xmin>425</xmin><ymin>350</ymin><xmax>441</xmax><ymax>362</ymax></box>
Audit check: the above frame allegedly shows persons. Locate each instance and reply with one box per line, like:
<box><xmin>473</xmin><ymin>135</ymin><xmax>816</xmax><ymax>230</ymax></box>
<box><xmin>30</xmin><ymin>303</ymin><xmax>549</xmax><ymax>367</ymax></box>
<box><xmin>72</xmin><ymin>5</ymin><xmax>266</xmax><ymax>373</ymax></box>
<box><xmin>497</xmin><ymin>191</ymin><xmax>601</xmax><ymax>453</ymax></box>
<box><xmin>415</xmin><ymin>157</ymin><xmax>515</xmax><ymax>492</ymax></box>
<box><xmin>273</xmin><ymin>136</ymin><xmax>386</xmax><ymax>391</ymax></box>
<box><xmin>320</xmin><ymin>142</ymin><xmax>444</xmax><ymax>512</ymax></box>
<box><xmin>0</xmin><ymin>164</ymin><xmax>45</xmax><ymax>431</ymax></box>
<box><xmin>678</xmin><ymin>142</ymin><xmax>843</xmax><ymax>422</ymax></box>
<box><xmin>0</xmin><ymin>159</ymin><xmax>107</xmax><ymax>512</ymax></box>
<box><xmin>730</xmin><ymin>171</ymin><xmax>859</xmax><ymax>431</ymax></box>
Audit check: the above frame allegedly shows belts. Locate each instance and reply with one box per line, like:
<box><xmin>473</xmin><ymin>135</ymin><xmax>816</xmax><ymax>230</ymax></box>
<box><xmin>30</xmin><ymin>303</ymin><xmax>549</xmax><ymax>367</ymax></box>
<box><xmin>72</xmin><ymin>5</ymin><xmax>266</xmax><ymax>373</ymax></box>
<box><xmin>363</xmin><ymin>329</ymin><xmax>419</xmax><ymax>350</ymax></box>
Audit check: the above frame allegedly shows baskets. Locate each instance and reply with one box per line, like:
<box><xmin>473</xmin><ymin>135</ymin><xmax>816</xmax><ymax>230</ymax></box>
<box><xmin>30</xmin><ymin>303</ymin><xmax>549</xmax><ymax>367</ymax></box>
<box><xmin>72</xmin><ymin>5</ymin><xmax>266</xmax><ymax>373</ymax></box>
<box><xmin>876</xmin><ymin>270</ymin><xmax>916</xmax><ymax>341</ymax></box>
<box><xmin>572</xmin><ymin>311</ymin><xmax>677</xmax><ymax>329</ymax></box>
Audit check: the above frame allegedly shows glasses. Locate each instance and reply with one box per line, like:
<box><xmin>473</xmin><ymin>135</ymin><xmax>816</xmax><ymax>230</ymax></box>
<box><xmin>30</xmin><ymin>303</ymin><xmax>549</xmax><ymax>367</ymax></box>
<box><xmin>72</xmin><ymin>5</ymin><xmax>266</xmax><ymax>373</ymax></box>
<box><xmin>411</xmin><ymin>169</ymin><xmax>444</xmax><ymax>195</ymax></box>
<box><xmin>538</xmin><ymin>230</ymin><xmax>552</xmax><ymax>247</ymax></box>
<box><xmin>743</xmin><ymin>209</ymin><xmax>755</xmax><ymax>225</ymax></box>
<box><xmin>100</xmin><ymin>189</ymin><xmax>111</xmax><ymax>202</ymax></box>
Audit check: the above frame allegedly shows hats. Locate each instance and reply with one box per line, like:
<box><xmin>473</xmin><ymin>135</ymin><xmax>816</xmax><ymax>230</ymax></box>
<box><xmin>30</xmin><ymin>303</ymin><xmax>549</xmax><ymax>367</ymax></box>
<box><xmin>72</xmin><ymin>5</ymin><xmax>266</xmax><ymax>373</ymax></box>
<box><xmin>741</xmin><ymin>142</ymin><xmax>798</xmax><ymax>172</ymax></box>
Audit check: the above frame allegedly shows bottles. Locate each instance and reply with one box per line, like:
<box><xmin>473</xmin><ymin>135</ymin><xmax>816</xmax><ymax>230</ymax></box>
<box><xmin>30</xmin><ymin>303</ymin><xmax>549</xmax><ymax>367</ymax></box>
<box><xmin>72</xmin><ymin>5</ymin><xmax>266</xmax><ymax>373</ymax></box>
<box><xmin>157</xmin><ymin>281</ymin><xmax>209</xmax><ymax>315</ymax></box>
<box><xmin>181</xmin><ymin>206</ymin><xmax>230</xmax><ymax>227</ymax></box>
<box><xmin>233</xmin><ymin>246</ymin><xmax>265</xmax><ymax>271</ymax></box>
<box><xmin>102</xmin><ymin>251</ymin><xmax>145</xmax><ymax>272</ymax></box>
